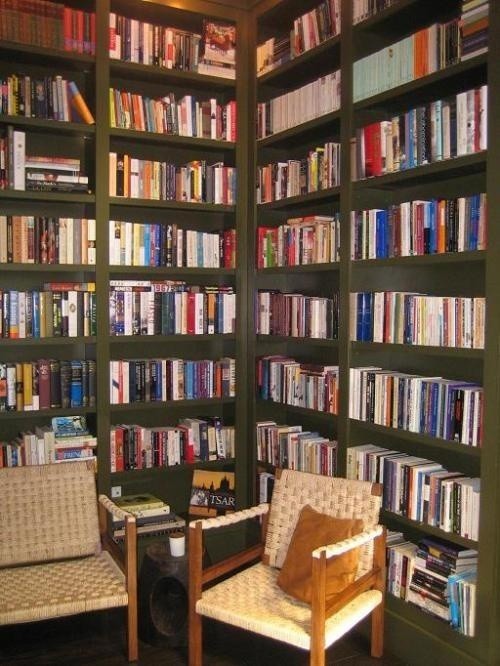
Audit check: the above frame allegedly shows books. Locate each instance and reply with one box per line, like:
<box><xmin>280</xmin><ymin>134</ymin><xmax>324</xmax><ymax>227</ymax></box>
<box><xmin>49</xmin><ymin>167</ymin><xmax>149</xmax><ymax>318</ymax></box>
<box><xmin>0</xmin><ymin>0</ymin><xmax>236</xmax><ymax>474</ymax></box>
<box><xmin>254</xmin><ymin>0</ymin><xmax>490</xmax><ymax>638</ymax></box>
<box><xmin>107</xmin><ymin>494</ymin><xmax>186</xmax><ymax>544</ymax></box>
<box><xmin>188</xmin><ymin>470</ymin><xmax>235</xmax><ymax>518</ymax></box>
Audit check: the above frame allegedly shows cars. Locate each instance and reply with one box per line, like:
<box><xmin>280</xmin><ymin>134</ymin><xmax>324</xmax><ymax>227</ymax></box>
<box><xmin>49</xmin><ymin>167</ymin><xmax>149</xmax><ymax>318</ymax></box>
<box><xmin>169</xmin><ymin>531</ymin><xmax>186</xmax><ymax>558</ymax></box>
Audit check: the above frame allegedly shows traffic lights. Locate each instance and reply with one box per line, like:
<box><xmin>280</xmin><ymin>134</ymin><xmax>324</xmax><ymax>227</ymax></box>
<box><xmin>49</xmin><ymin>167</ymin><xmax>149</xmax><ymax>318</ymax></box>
<box><xmin>277</xmin><ymin>502</ymin><xmax>365</xmax><ymax>608</ymax></box>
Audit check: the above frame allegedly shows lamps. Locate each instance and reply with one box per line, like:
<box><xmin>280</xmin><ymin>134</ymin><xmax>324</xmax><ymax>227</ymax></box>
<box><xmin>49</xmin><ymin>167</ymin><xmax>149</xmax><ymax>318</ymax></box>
<box><xmin>187</xmin><ymin>465</ymin><xmax>385</xmax><ymax>666</ymax></box>
<box><xmin>0</xmin><ymin>456</ymin><xmax>141</xmax><ymax>663</ymax></box>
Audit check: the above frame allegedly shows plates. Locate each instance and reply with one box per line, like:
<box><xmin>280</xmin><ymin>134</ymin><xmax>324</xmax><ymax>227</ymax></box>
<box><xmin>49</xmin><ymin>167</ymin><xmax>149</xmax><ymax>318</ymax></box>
<box><xmin>0</xmin><ymin>0</ymin><xmax>248</xmax><ymax>578</ymax></box>
<box><xmin>247</xmin><ymin>0</ymin><xmax>500</xmax><ymax>665</ymax></box>
